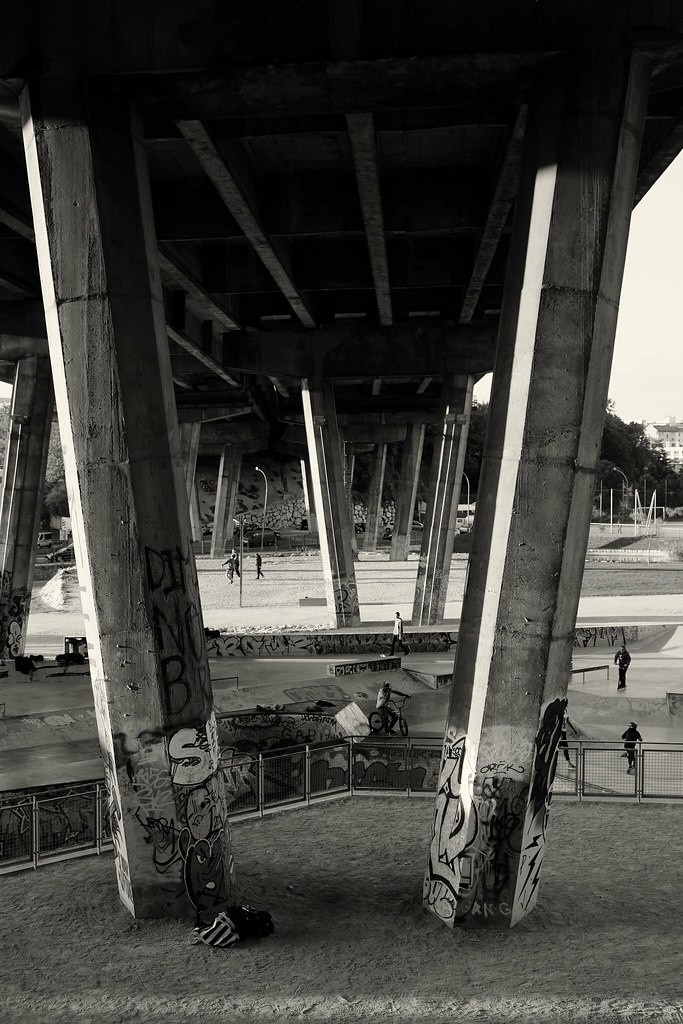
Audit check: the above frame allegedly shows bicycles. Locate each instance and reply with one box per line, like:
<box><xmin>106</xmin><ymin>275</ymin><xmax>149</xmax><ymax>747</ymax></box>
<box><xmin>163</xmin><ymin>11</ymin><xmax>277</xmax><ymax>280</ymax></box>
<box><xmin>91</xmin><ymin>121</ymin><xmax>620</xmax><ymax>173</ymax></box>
<box><xmin>368</xmin><ymin>695</ymin><xmax>411</xmax><ymax>736</ymax></box>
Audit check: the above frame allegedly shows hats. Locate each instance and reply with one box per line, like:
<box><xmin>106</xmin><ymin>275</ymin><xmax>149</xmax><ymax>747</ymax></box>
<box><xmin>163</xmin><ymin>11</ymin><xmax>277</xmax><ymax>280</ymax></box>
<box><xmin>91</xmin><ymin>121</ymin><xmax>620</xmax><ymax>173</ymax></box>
<box><xmin>383</xmin><ymin>681</ymin><xmax>391</xmax><ymax>687</ymax></box>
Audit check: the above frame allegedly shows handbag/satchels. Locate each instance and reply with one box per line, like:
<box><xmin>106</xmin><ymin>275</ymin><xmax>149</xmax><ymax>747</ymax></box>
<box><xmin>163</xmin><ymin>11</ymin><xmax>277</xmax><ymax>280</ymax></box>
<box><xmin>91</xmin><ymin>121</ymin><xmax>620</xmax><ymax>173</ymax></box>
<box><xmin>226</xmin><ymin>906</ymin><xmax>275</xmax><ymax>940</ymax></box>
<box><xmin>198</xmin><ymin>911</ymin><xmax>240</xmax><ymax>948</ymax></box>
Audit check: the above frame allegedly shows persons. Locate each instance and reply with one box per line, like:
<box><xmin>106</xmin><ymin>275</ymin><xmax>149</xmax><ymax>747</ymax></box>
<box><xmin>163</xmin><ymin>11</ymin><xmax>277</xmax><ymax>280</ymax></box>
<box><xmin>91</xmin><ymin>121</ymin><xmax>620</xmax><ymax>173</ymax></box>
<box><xmin>221</xmin><ymin>547</ymin><xmax>265</xmax><ymax>585</ymax></box>
<box><xmin>375</xmin><ymin>680</ymin><xmax>412</xmax><ymax>736</ymax></box>
<box><xmin>558</xmin><ymin>709</ymin><xmax>577</xmax><ymax>770</ymax></box>
<box><xmin>614</xmin><ymin>646</ymin><xmax>631</xmax><ymax>689</ymax></box>
<box><xmin>387</xmin><ymin>612</ymin><xmax>410</xmax><ymax>657</ymax></box>
<box><xmin>620</xmin><ymin>722</ymin><xmax>642</xmax><ymax>769</ymax></box>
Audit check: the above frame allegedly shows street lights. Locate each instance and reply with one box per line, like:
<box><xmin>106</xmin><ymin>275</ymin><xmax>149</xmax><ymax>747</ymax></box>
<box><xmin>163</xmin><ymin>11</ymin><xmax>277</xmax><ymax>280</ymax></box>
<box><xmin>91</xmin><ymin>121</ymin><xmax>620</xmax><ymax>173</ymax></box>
<box><xmin>255</xmin><ymin>467</ymin><xmax>267</xmax><ymax>552</ymax></box>
<box><xmin>613</xmin><ymin>468</ymin><xmax>628</xmax><ymax>509</ymax></box>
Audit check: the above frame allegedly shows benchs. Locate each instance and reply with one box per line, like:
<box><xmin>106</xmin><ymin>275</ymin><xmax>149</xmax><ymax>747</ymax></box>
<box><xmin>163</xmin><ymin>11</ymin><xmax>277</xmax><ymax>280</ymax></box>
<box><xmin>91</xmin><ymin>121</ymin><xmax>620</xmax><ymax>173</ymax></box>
<box><xmin>14</xmin><ymin>652</ymin><xmax>89</xmax><ymax>682</ymax></box>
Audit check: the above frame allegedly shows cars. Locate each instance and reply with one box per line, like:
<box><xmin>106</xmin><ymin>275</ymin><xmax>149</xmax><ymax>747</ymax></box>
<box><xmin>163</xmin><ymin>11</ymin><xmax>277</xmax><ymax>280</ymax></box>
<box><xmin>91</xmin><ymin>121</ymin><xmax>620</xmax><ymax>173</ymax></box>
<box><xmin>243</xmin><ymin>530</ymin><xmax>275</xmax><ymax>546</ymax></box>
<box><xmin>355</xmin><ymin>523</ymin><xmax>366</xmax><ymax>532</ymax></box>
<box><xmin>355</xmin><ymin>525</ymin><xmax>363</xmax><ymax>533</ymax></box>
<box><xmin>45</xmin><ymin>548</ymin><xmax>75</xmax><ymax>562</ymax></box>
<box><xmin>36</xmin><ymin>532</ymin><xmax>53</xmax><ymax>549</ymax></box>
<box><xmin>454</xmin><ymin>518</ymin><xmax>473</xmax><ymax>537</ymax></box>
<box><xmin>384</xmin><ymin>522</ymin><xmax>394</xmax><ymax>532</ymax></box>
<box><xmin>411</xmin><ymin>521</ymin><xmax>424</xmax><ymax>531</ymax></box>
<box><xmin>237</xmin><ymin>524</ymin><xmax>258</xmax><ymax>538</ymax></box>
<box><xmin>202</xmin><ymin>524</ymin><xmax>209</xmax><ymax>534</ymax></box>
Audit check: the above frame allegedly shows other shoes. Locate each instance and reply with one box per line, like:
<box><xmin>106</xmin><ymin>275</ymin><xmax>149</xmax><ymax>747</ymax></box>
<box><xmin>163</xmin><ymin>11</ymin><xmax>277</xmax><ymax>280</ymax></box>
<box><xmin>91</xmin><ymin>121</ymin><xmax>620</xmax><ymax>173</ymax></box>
<box><xmin>388</xmin><ymin>653</ymin><xmax>394</xmax><ymax>657</ymax></box>
<box><xmin>385</xmin><ymin>732</ymin><xmax>390</xmax><ymax>736</ymax></box>
<box><xmin>389</xmin><ymin>729</ymin><xmax>396</xmax><ymax>733</ymax></box>
<box><xmin>568</xmin><ymin>764</ymin><xmax>578</xmax><ymax>770</ymax></box>
<box><xmin>621</xmin><ymin>753</ymin><xmax>627</xmax><ymax>758</ymax></box>
<box><xmin>404</xmin><ymin>651</ymin><xmax>410</xmax><ymax>655</ymax></box>
<box><xmin>627</xmin><ymin>766</ymin><xmax>632</xmax><ymax>773</ymax></box>
